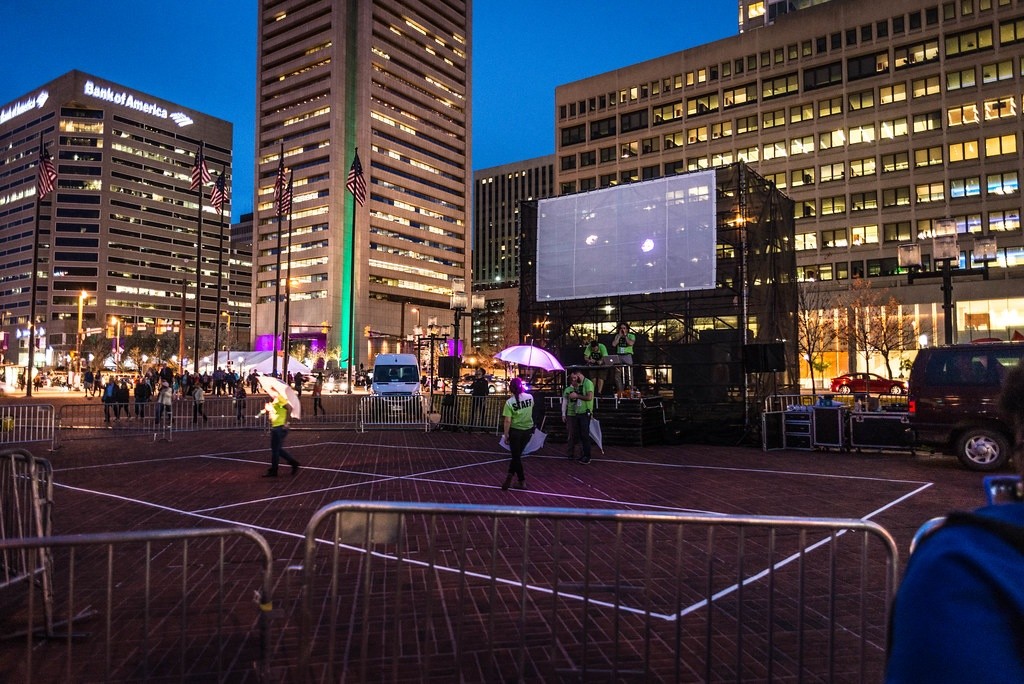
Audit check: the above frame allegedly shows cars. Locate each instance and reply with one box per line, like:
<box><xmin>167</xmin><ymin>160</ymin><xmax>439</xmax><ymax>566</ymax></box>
<box><xmin>423</xmin><ymin>372</ymin><xmax>564</xmax><ymax>395</ymax></box>
<box><xmin>830</xmin><ymin>372</ymin><xmax>909</xmax><ymax>396</ymax></box>
<box><xmin>293</xmin><ymin>367</ymin><xmax>374</xmax><ymax>392</ymax></box>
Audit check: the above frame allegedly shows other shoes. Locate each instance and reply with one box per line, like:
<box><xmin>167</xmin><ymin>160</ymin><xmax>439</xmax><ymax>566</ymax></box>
<box><xmin>261</xmin><ymin>472</ymin><xmax>279</xmax><ymax>477</ymax></box>
<box><xmin>578</xmin><ymin>456</ymin><xmax>591</xmax><ymax>464</ymax></box>
<box><xmin>290</xmin><ymin>461</ymin><xmax>301</xmax><ymax>476</ymax></box>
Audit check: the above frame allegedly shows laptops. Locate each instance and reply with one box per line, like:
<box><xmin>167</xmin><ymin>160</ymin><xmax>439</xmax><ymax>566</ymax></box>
<box><xmin>604</xmin><ymin>355</ymin><xmax>625</xmax><ymax>365</ymax></box>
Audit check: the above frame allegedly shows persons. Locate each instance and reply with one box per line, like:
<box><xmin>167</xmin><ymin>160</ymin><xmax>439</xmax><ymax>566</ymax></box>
<box><xmin>562</xmin><ymin>371</ymin><xmax>594</xmax><ymax>459</ymax></box>
<box><xmin>467</xmin><ymin>368</ymin><xmax>489</xmax><ymax>426</ymax></box>
<box><xmin>884</xmin><ymin>361</ymin><xmax>1024</xmax><ymax>684</ymax></box>
<box><xmin>261</xmin><ymin>387</ymin><xmax>299</xmax><ymax>477</ymax></box>
<box><xmin>500</xmin><ymin>378</ymin><xmax>534</xmax><ymax>491</ymax></box>
<box><xmin>20</xmin><ymin>363</ymin><xmax>438</xmax><ymax>424</ymax></box>
<box><xmin>584</xmin><ymin>341</ymin><xmax>609</xmax><ymax>393</ymax></box>
<box><xmin>612</xmin><ymin>322</ymin><xmax>635</xmax><ymax>391</ymax></box>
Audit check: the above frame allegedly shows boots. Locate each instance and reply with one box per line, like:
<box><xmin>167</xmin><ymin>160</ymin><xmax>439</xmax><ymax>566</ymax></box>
<box><xmin>519</xmin><ymin>474</ymin><xmax>528</xmax><ymax>489</ymax></box>
<box><xmin>502</xmin><ymin>474</ymin><xmax>513</xmax><ymax>490</ymax></box>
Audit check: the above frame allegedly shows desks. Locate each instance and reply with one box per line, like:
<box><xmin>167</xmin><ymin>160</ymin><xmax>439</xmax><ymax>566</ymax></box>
<box><xmin>563</xmin><ymin>364</ymin><xmax>631</xmax><ymax>397</ymax></box>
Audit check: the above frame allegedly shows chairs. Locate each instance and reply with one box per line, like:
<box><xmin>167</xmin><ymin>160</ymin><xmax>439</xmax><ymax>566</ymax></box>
<box><xmin>728</xmin><ymin>403</ymin><xmax>762</xmax><ymax>448</ymax></box>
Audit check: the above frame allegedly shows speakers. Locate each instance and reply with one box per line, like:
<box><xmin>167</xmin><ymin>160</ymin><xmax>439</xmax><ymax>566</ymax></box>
<box><xmin>438</xmin><ymin>357</ymin><xmax>459</xmax><ymax>379</ymax></box>
<box><xmin>744</xmin><ymin>342</ymin><xmax>786</xmax><ymax>374</ymax></box>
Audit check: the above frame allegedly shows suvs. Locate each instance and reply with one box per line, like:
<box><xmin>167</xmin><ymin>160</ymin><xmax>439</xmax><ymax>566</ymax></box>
<box><xmin>903</xmin><ymin>341</ymin><xmax>1024</xmax><ymax>473</ymax></box>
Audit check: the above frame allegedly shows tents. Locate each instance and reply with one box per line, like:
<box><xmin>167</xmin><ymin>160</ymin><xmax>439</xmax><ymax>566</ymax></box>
<box><xmin>192</xmin><ymin>350</ymin><xmax>312</xmax><ymax>377</ymax></box>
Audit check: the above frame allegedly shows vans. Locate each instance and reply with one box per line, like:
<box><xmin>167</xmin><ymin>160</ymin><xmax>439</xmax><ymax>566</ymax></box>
<box><xmin>368</xmin><ymin>353</ymin><xmax>427</xmax><ymax>415</ymax></box>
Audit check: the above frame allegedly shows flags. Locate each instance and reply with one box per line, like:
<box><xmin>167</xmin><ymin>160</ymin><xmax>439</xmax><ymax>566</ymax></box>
<box><xmin>274</xmin><ymin>156</ymin><xmax>293</xmax><ymax>216</ymax></box>
<box><xmin>190</xmin><ymin>147</ymin><xmax>227</xmax><ymax>214</ymax></box>
<box><xmin>38</xmin><ymin>140</ymin><xmax>58</xmax><ymax>199</ymax></box>
<box><xmin>346</xmin><ymin>154</ymin><xmax>367</xmax><ymax>207</ymax></box>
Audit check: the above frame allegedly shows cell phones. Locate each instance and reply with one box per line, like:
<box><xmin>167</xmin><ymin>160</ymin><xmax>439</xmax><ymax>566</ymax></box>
<box><xmin>983</xmin><ymin>475</ymin><xmax>1024</xmax><ymax>506</ymax></box>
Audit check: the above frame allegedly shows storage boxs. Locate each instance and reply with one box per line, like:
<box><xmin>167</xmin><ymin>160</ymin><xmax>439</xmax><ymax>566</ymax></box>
<box><xmin>812</xmin><ymin>406</ymin><xmax>846</xmax><ymax>449</ymax></box>
<box><xmin>783</xmin><ymin>412</ymin><xmax>814</xmax><ymax>451</ymax></box>
<box><xmin>848</xmin><ymin>411</ymin><xmax>918</xmax><ymax>450</ymax></box>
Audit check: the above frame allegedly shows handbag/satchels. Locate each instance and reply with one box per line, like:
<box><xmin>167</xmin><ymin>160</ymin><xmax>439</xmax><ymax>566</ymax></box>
<box><xmin>232</xmin><ymin>389</ymin><xmax>237</xmax><ymax>406</ymax></box>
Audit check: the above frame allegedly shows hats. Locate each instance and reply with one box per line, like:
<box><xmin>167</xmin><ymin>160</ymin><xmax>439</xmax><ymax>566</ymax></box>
<box><xmin>162</xmin><ymin>382</ymin><xmax>168</xmax><ymax>387</ymax></box>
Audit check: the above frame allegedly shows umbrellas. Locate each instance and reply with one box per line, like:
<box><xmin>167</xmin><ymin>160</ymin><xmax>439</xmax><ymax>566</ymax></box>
<box><xmin>494</xmin><ymin>339</ymin><xmax>564</xmax><ymax>388</ymax></box>
<box><xmin>499</xmin><ymin>427</ymin><xmax>548</xmax><ymax>455</ymax></box>
<box><xmin>589</xmin><ymin>418</ymin><xmax>604</xmax><ymax>455</ymax></box>
<box><xmin>254</xmin><ymin>375</ymin><xmax>301</xmax><ymax>420</ymax></box>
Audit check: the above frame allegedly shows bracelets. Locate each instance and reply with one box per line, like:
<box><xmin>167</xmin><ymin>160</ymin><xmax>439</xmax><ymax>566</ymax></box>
<box><xmin>562</xmin><ymin>412</ymin><xmax>566</xmax><ymax>416</ymax></box>
<box><xmin>624</xmin><ymin>335</ymin><xmax>627</xmax><ymax>337</ymax></box>
<box><xmin>287</xmin><ymin>422</ymin><xmax>290</xmax><ymax>425</ymax></box>
